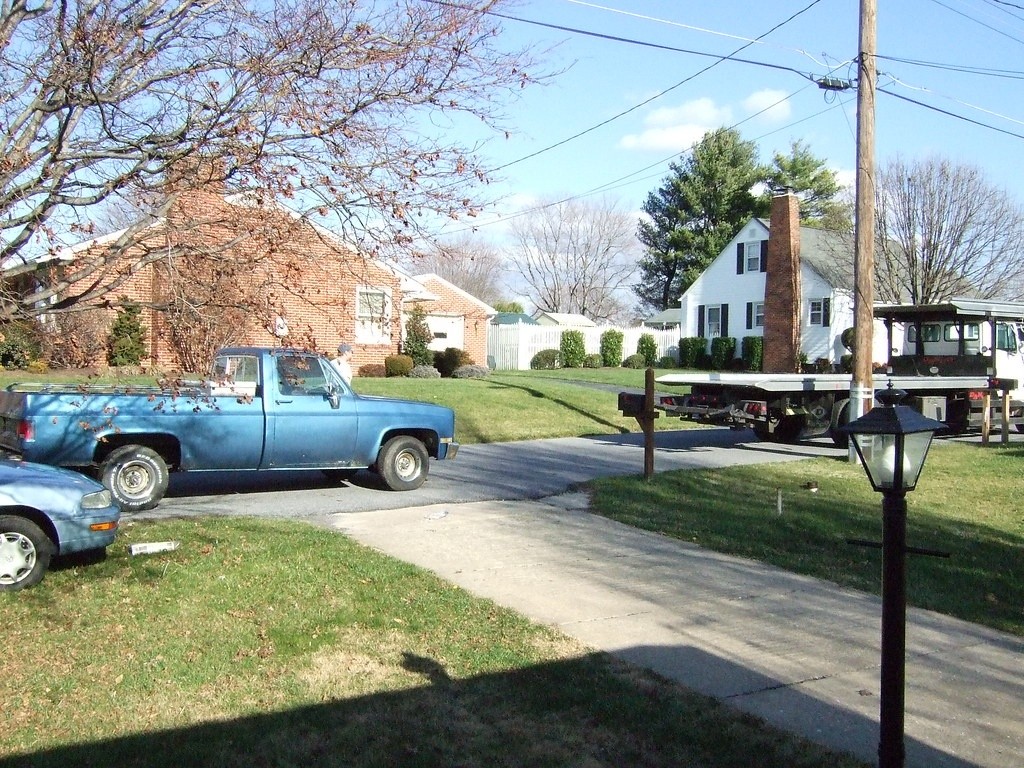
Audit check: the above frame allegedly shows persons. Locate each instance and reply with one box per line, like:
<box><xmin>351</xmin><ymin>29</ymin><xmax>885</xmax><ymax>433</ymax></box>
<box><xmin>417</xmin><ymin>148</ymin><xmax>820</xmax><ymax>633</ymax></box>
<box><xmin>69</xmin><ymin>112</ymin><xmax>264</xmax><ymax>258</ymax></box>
<box><xmin>325</xmin><ymin>343</ymin><xmax>355</xmax><ymax>390</ymax></box>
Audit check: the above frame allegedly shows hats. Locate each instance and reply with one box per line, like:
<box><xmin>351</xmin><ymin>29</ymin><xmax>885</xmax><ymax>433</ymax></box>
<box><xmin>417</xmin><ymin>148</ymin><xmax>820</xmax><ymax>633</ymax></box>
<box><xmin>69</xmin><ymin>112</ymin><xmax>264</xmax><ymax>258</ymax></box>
<box><xmin>337</xmin><ymin>344</ymin><xmax>352</xmax><ymax>354</ymax></box>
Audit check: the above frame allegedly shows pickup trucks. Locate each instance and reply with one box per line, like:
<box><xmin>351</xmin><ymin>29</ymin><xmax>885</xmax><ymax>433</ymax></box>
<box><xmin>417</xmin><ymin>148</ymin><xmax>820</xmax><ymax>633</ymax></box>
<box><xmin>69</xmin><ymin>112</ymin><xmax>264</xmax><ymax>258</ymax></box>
<box><xmin>0</xmin><ymin>348</ymin><xmax>461</xmax><ymax>512</ymax></box>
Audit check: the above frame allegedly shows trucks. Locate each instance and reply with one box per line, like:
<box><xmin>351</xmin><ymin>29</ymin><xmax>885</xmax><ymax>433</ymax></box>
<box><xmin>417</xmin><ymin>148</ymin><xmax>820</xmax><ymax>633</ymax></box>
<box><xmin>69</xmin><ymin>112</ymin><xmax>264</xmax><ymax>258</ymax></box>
<box><xmin>652</xmin><ymin>298</ymin><xmax>1023</xmax><ymax>446</ymax></box>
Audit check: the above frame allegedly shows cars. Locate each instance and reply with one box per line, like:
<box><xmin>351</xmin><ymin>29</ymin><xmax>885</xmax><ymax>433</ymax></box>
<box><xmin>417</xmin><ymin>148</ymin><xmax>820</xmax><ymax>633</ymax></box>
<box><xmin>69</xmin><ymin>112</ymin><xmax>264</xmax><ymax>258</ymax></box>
<box><xmin>0</xmin><ymin>450</ymin><xmax>123</xmax><ymax>593</ymax></box>
